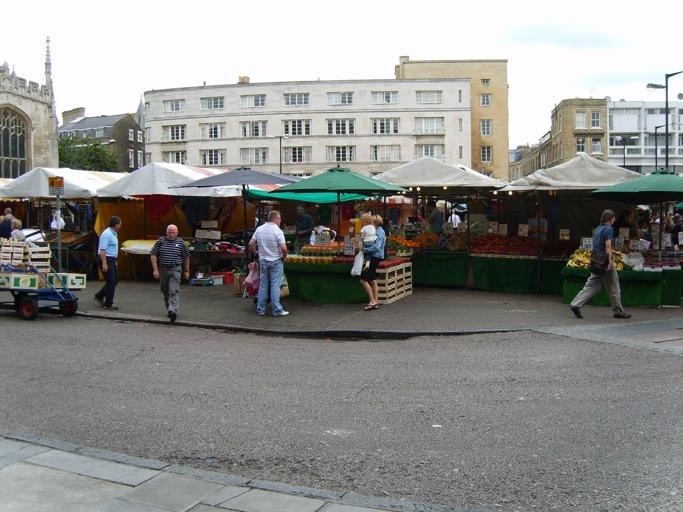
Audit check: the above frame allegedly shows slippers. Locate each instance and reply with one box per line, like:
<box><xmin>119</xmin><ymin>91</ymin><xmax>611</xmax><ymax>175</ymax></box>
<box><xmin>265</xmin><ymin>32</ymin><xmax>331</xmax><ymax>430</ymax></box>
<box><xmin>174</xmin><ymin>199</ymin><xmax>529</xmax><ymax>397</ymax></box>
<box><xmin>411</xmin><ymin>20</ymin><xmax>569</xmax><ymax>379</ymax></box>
<box><xmin>364</xmin><ymin>303</ymin><xmax>379</xmax><ymax>310</ymax></box>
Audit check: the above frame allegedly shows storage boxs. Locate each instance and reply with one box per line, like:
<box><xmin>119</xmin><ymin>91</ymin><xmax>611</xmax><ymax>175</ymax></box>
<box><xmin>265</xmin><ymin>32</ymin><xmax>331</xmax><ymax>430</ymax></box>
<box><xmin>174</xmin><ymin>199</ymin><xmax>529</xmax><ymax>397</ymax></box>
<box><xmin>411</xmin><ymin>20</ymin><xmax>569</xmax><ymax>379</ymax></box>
<box><xmin>46</xmin><ymin>273</ymin><xmax>86</xmax><ymax>289</ymax></box>
<box><xmin>187</xmin><ymin>269</ymin><xmax>246</xmax><ymax>291</ymax></box>
<box><xmin>0</xmin><ymin>236</ymin><xmax>52</xmax><ymax>287</ymax></box>
<box><xmin>0</xmin><ymin>273</ymin><xmax>38</xmax><ymax>292</ymax></box>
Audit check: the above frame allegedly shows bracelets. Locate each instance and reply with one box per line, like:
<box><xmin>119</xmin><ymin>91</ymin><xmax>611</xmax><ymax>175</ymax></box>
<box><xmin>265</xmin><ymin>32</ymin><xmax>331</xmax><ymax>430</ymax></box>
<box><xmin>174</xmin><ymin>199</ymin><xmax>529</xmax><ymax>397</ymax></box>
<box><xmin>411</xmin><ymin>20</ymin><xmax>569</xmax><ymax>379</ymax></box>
<box><xmin>186</xmin><ymin>268</ymin><xmax>189</xmax><ymax>272</ymax></box>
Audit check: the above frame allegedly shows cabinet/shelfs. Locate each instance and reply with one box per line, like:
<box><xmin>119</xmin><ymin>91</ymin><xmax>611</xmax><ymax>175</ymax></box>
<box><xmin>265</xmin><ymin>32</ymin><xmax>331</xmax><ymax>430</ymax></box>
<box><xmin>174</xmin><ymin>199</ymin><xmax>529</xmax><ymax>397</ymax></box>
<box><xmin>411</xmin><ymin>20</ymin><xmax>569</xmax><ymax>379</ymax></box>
<box><xmin>372</xmin><ymin>261</ymin><xmax>412</xmax><ymax>304</ymax></box>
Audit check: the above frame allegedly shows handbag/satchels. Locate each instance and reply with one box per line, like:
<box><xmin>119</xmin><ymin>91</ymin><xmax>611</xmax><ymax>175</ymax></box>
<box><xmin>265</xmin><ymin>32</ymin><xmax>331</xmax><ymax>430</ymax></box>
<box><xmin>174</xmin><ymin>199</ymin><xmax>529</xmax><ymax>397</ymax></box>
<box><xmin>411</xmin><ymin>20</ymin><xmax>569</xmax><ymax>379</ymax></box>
<box><xmin>350</xmin><ymin>251</ymin><xmax>364</xmax><ymax>276</ymax></box>
<box><xmin>590</xmin><ymin>252</ymin><xmax>605</xmax><ymax>275</ymax></box>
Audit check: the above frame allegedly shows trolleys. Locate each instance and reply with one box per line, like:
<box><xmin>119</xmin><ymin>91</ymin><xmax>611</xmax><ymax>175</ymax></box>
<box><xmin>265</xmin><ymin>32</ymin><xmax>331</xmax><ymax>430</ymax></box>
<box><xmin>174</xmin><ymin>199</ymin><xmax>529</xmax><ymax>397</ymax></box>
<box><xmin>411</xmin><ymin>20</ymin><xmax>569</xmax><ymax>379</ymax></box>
<box><xmin>0</xmin><ymin>261</ymin><xmax>82</xmax><ymax>321</ymax></box>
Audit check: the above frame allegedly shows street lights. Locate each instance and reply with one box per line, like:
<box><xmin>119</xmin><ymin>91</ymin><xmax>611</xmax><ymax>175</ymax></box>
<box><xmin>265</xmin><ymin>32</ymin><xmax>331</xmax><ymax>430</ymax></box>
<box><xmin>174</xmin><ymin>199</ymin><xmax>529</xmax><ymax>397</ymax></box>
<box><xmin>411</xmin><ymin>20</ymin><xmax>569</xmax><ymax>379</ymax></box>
<box><xmin>621</xmin><ymin>135</ymin><xmax>639</xmax><ymax>168</ymax></box>
<box><xmin>654</xmin><ymin>122</ymin><xmax>683</xmax><ymax>171</ymax></box>
<box><xmin>646</xmin><ymin>72</ymin><xmax>683</xmax><ymax>171</ymax></box>
<box><xmin>280</xmin><ymin>135</ymin><xmax>289</xmax><ymax>173</ymax></box>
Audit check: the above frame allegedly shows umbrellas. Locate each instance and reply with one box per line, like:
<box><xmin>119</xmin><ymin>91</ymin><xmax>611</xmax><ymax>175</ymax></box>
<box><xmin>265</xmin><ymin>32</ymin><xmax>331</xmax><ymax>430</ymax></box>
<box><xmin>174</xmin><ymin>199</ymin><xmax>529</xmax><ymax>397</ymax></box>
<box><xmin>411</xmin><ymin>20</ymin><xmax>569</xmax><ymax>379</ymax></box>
<box><xmin>269</xmin><ymin>164</ymin><xmax>410</xmax><ymax>255</ymax></box>
<box><xmin>167</xmin><ymin>165</ymin><xmax>304</xmax><ymax>276</ymax></box>
<box><xmin>592</xmin><ymin>168</ymin><xmax>683</xmax><ymax>260</ymax></box>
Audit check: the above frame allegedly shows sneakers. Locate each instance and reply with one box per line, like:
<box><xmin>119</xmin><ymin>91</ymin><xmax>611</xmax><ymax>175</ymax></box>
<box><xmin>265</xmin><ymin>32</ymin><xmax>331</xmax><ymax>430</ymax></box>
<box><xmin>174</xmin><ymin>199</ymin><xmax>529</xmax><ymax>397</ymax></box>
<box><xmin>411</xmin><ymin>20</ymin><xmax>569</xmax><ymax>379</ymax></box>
<box><xmin>170</xmin><ymin>311</ymin><xmax>176</xmax><ymax>325</ymax></box>
<box><xmin>614</xmin><ymin>312</ymin><xmax>631</xmax><ymax>318</ymax></box>
<box><xmin>91</xmin><ymin>296</ymin><xmax>119</xmax><ymax>309</ymax></box>
<box><xmin>257</xmin><ymin>309</ymin><xmax>290</xmax><ymax>316</ymax></box>
<box><xmin>571</xmin><ymin>306</ymin><xmax>583</xmax><ymax>318</ymax></box>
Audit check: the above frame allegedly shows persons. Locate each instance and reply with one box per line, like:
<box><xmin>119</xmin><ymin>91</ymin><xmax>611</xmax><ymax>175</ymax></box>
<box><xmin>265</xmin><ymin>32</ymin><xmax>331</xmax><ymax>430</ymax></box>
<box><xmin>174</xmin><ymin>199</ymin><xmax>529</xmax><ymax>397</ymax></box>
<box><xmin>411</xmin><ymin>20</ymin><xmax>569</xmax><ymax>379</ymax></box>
<box><xmin>448</xmin><ymin>209</ymin><xmax>461</xmax><ymax>231</ymax></box>
<box><xmin>360</xmin><ymin>213</ymin><xmax>377</xmax><ymax>271</ymax></box>
<box><xmin>570</xmin><ymin>209</ymin><xmax>632</xmax><ymax>318</ymax></box>
<box><xmin>151</xmin><ymin>223</ymin><xmax>191</xmax><ymax>324</ymax></box>
<box><xmin>249</xmin><ymin>210</ymin><xmax>289</xmax><ymax>317</ymax></box>
<box><xmin>92</xmin><ymin>216</ymin><xmax>122</xmax><ymax>310</ymax></box>
<box><xmin>528</xmin><ymin>207</ymin><xmax>549</xmax><ymax>240</ymax></box>
<box><xmin>618</xmin><ymin>208</ymin><xmax>683</xmax><ymax>248</ymax></box>
<box><xmin>419</xmin><ymin>200</ymin><xmax>443</xmax><ymax>236</ymax></box>
<box><xmin>359</xmin><ymin>214</ymin><xmax>387</xmax><ymax>310</ymax></box>
<box><xmin>0</xmin><ymin>207</ymin><xmax>22</xmax><ymax>239</ymax></box>
<box><xmin>294</xmin><ymin>204</ymin><xmax>315</xmax><ymax>254</ymax></box>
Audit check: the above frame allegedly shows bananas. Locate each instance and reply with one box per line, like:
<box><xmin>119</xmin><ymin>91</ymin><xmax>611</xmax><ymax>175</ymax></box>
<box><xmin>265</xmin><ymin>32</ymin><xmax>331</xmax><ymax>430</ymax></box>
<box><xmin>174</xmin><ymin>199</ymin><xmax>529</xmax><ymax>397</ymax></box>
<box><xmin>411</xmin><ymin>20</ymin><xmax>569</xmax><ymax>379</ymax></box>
<box><xmin>567</xmin><ymin>249</ymin><xmax>624</xmax><ymax>272</ymax></box>
